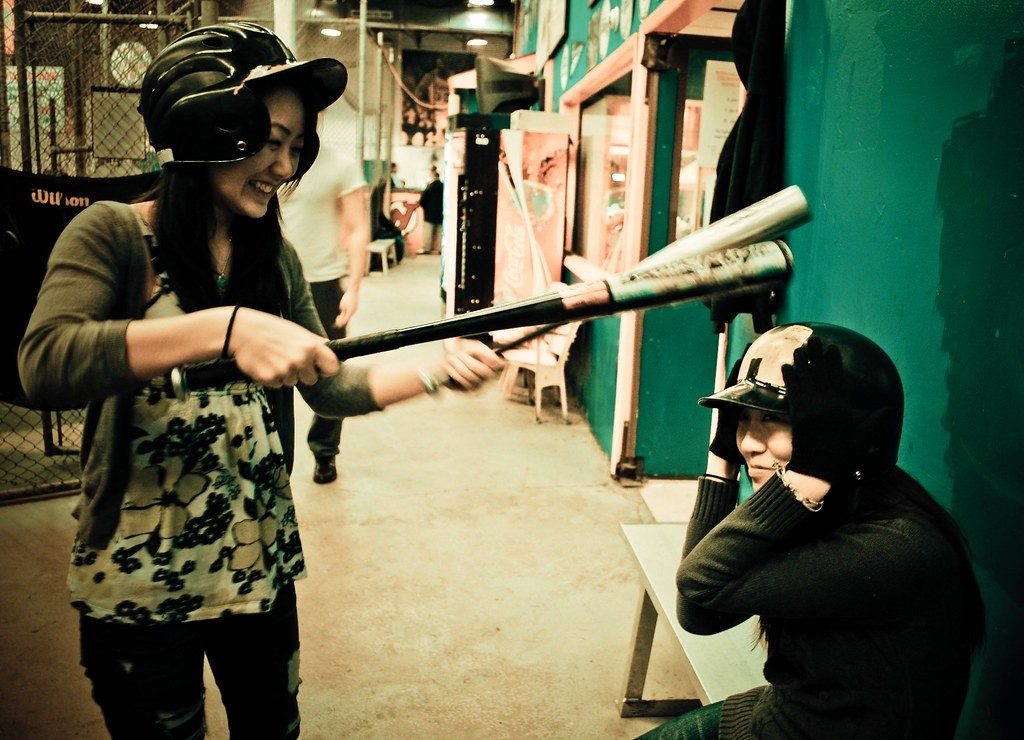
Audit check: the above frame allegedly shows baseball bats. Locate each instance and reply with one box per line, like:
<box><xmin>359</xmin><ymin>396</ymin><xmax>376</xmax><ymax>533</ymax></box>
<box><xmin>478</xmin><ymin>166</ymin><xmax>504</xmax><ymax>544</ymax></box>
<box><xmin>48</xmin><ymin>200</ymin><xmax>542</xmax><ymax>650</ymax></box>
<box><xmin>419</xmin><ymin>183</ymin><xmax>814</xmax><ymax>396</ymax></box>
<box><xmin>171</xmin><ymin>237</ymin><xmax>794</xmax><ymax>405</ymax></box>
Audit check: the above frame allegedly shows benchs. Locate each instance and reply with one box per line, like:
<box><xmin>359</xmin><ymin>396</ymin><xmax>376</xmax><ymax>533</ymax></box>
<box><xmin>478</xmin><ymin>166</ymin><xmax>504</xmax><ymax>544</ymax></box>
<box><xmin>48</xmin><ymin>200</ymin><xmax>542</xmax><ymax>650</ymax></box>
<box><xmin>365</xmin><ymin>239</ymin><xmax>397</xmax><ymax>276</ymax></box>
<box><xmin>615</xmin><ymin>520</ymin><xmax>772</xmax><ymax>717</ymax></box>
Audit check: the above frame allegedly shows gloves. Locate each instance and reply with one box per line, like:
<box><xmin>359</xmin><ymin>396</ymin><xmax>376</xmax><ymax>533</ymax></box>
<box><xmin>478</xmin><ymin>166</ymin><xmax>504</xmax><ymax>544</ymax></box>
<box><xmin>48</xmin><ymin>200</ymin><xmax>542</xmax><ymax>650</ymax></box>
<box><xmin>709</xmin><ymin>342</ymin><xmax>752</xmax><ymax>464</ymax></box>
<box><xmin>782</xmin><ymin>338</ymin><xmax>893</xmax><ymax>484</ymax></box>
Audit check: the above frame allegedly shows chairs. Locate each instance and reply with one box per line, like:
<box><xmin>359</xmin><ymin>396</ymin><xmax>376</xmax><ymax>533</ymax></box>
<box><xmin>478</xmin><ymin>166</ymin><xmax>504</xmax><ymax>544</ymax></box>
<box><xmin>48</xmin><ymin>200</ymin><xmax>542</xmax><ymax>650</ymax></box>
<box><xmin>488</xmin><ymin>281</ymin><xmax>585</xmax><ymax>423</ymax></box>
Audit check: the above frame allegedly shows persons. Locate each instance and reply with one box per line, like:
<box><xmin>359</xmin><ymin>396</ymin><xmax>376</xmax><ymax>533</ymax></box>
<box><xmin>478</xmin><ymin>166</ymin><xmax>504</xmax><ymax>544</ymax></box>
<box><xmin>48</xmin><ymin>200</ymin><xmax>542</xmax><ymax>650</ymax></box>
<box><xmin>271</xmin><ymin>128</ymin><xmax>371</xmax><ymax>484</ymax></box>
<box><xmin>16</xmin><ymin>19</ymin><xmax>510</xmax><ymax>739</ymax></box>
<box><xmin>414</xmin><ymin>171</ymin><xmax>443</xmax><ymax>255</ymax></box>
<box><xmin>411</xmin><ymin>57</ymin><xmax>462</xmax><ymax>146</ymax></box>
<box><xmin>389</xmin><ymin>162</ymin><xmax>443</xmax><ymax>190</ymax></box>
<box><xmin>633</xmin><ymin>323</ymin><xmax>982</xmax><ymax>740</ymax></box>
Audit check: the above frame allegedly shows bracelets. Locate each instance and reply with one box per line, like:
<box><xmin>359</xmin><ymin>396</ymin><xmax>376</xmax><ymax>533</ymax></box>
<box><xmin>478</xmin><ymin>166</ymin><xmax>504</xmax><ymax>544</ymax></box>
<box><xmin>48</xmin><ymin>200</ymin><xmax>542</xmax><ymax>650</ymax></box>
<box><xmin>419</xmin><ymin>357</ymin><xmax>440</xmax><ymax>395</ymax></box>
<box><xmin>772</xmin><ymin>461</ymin><xmax>826</xmax><ymax>513</ymax></box>
<box><xmin>220</xmin><ymin>305</ymin><xmax>242</xmax><ymax>360</ymax></box>
<box><xmin>703</xmin><ymin>471</ymin><xmax>739</xmax><ymax>484</ymax></box>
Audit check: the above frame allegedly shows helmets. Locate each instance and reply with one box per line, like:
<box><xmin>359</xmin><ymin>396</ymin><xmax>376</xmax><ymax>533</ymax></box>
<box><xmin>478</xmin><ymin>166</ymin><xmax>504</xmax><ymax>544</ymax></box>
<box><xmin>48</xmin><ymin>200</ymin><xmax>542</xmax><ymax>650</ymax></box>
<box><xmin>698</xmin><ymin>321</ymin><xmax>904</xmax><ymax>458</ymax></box>
<box><xmin>136</xmin><ymin>22</ymin><xmax>348</xmax><ymax>165</ymax></box>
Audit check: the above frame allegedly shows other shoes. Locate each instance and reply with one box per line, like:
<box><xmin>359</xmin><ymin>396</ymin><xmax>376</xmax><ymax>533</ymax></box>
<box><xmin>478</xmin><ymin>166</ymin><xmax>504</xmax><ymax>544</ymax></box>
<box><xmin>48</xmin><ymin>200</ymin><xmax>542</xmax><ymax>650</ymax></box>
<box><xmin>313</xmin><ymin>460</ymin><xmax>337</xmax><ymax>482</ymax></box>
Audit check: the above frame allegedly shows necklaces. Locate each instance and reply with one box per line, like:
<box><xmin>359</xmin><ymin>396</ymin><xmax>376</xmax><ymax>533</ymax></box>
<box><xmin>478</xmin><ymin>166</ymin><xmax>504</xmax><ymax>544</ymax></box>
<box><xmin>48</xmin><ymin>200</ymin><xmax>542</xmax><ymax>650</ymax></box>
<box><xmin>214</xmin><ymin>233</ymin><xmax>232</xmax><ymax>289</ymax></box>
